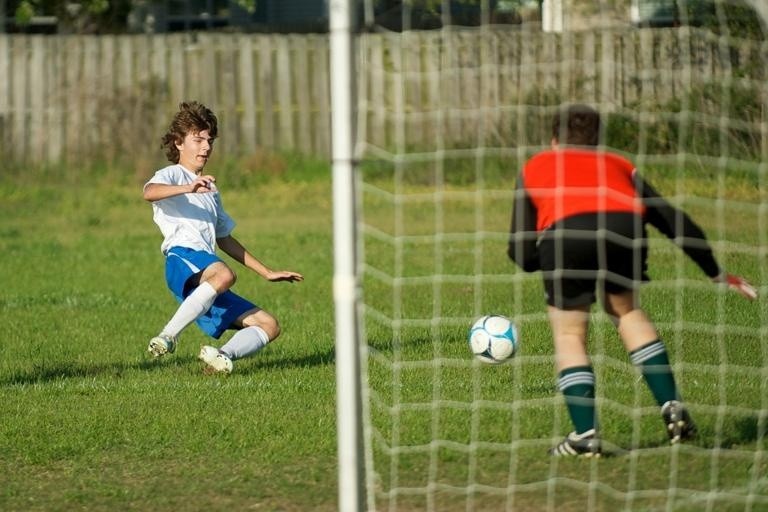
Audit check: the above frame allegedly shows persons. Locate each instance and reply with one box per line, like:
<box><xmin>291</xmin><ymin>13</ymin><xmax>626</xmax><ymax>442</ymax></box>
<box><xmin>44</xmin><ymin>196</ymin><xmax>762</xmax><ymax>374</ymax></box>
<box><xmin>508</xmin><ymin>101</ymin><xmax>757</xmax><ymax>462</ymax></box>
<box><xmin>142</xmin><ymin>101</ymin><xmax>305</xmax><ymax>377</ymax></box>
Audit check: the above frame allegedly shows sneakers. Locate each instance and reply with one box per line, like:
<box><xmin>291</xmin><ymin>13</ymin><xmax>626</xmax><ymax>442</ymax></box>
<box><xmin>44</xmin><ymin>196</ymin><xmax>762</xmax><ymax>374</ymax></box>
<box><xmin>661</xmin><ymin>404</ymin><xmax>701</xmax><ymax>446</ymax></box>
<box><xmin>199</xmin><ymin>345</ymin><xmax>234</xmax><ymax>375</ymax></box>
<box><xmin>147</xmin><ymin>332</ymin><xmax>177</xmax><ymax>357</ymax></box>
<box><xmin>545</xmin><ymin>428</ymin><xmax>603</xmax><ymax>460</ymax></box>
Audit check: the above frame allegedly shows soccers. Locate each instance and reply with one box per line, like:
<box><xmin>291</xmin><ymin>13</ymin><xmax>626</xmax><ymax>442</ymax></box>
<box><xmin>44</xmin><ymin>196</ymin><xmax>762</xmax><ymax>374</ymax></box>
<box><xmin>468</xmin><ymin>314</ymin><xmax>518</xmax><ymax>364</ymax></box>
